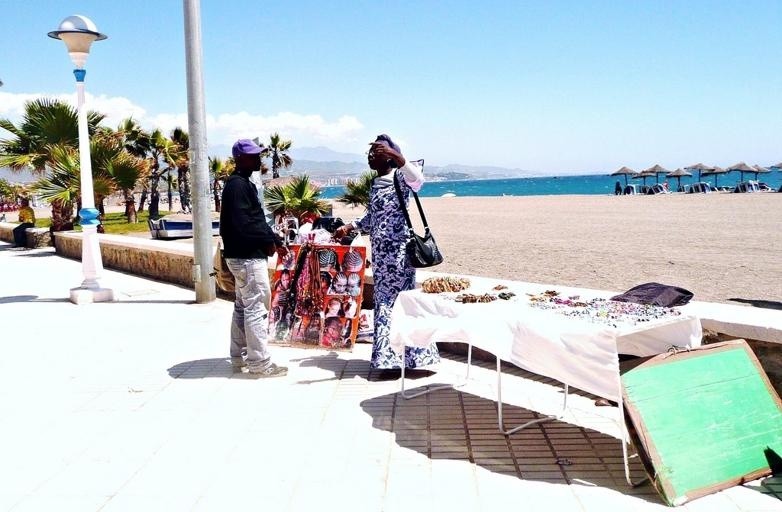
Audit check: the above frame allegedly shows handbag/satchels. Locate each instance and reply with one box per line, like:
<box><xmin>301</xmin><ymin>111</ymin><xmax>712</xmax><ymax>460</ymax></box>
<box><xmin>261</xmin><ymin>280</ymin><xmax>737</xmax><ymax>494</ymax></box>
<box><xmin>212</xmin><ymin>243</ymin><xmax>236</xmax><ymax>293</ymax></box>
<box><xmin>410</xmin><ymin>228</ymin><xmax>442</xmax><ymax>268</ymax></box>
<box><xmin>614</xmin><ymin>281</ymin><xmax>694</xmax><ymax>307</ymax></box>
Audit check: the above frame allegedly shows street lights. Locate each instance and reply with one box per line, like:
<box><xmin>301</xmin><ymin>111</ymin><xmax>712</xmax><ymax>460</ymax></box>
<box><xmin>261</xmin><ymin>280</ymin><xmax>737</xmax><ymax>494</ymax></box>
<box><xmin>46</xmin><ymin>10</ymin><xmax>117</xmax><ymax>307</ymax></box>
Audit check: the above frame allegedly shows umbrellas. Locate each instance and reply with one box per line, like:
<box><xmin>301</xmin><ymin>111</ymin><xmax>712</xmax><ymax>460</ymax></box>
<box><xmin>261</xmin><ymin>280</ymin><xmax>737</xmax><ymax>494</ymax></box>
<box><xmin>611</xmin><ymin>167</ymin><xmax>639</xmax><ymax>185</ymax></box>
<box><xmin>752</xmin><ymin>164</ymin><xmax>770</xmax><ymax>182</ymax></box>
<box><xmin>644</xmin><ymin>164</ymin><xmax>672</xmax><ymax>184</ymax></box>
<box><xmin>633</xmin><ymin>169</ymin><xmax>657</xmax><ymax>185</ymax></box>
<box><xmin>702</xmin><ymin>165</ymin><xmax>728</xmax><ymax>188</ymax></box>
<box><xmin>685</xmin><ymin>162</ymin><xmax>716</xmax><ymax>182</ymax></box>
<box><xmin>725</xmin><ymin>162</ymin><xmax>759</xmax><ymax>183</ymax></box>
<box><xmin>666</xmin><ymin>168</ymin><xmax>693</xmax><ymax>191</ymax></box>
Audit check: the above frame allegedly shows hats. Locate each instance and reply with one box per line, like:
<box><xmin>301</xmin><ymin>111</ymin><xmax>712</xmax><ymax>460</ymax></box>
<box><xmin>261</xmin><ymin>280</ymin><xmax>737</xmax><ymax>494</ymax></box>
<box><xmin>231</xmin><ymin>139</ymin><xmax>269</xmax><ymax>156</ymax></box>
<box><xmin>375</xmin><ymin>134</ymin><xmax>402</xmax><ymax>168</ymax></box>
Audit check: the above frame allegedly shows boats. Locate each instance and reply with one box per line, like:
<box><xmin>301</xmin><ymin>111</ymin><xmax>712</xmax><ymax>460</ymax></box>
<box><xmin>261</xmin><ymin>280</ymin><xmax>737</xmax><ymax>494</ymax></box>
<box><xmin>147</xmin><ymin>217</ymin><xmax>221</xmax><ymax>240</ymax></box>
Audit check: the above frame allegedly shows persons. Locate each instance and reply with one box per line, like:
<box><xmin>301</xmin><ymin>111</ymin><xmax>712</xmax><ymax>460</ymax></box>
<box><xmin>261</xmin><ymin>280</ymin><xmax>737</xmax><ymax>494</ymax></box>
<box><xmin>330</xmin><ymin>134</ymin><xmax>440</xmax><ymax>380</ymax></box>
<box><xmin>615</xmin><ymin>179</ymin><xmax>621</xmax><ymax>195</ymax></box>
<box><xmin>219</xmin><ymin>139</ymin><xmax>289</xmax><ymax>377</ymax></box>
<box><xmin>269</xmin><ymin>246</ymin><xmax>364</xmax><ymax>350</ymax></box>
<box><xmin>12</xmin><ymin>198</ymin><xmax>36</xmax><ymax>248</ymax></box>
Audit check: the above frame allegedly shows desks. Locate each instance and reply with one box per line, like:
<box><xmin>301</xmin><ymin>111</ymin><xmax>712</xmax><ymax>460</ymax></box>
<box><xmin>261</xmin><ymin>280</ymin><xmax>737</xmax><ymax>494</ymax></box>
<box><xmin>386</xmin><ymin>278</ymin><xmax>702</xmax><ymax>488</ymax></box>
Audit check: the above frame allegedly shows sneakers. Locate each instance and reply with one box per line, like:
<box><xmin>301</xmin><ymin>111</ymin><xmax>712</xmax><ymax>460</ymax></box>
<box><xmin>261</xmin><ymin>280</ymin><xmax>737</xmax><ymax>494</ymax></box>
<box><xmin>233</xmin><ymin>359</ymin><xmax>288</xmax><ymax>379</ymax></box>
<box><xmin>379</xmin><ymin>368</ymin><xmax>434</xmax><ymax>380</ymax></box>
